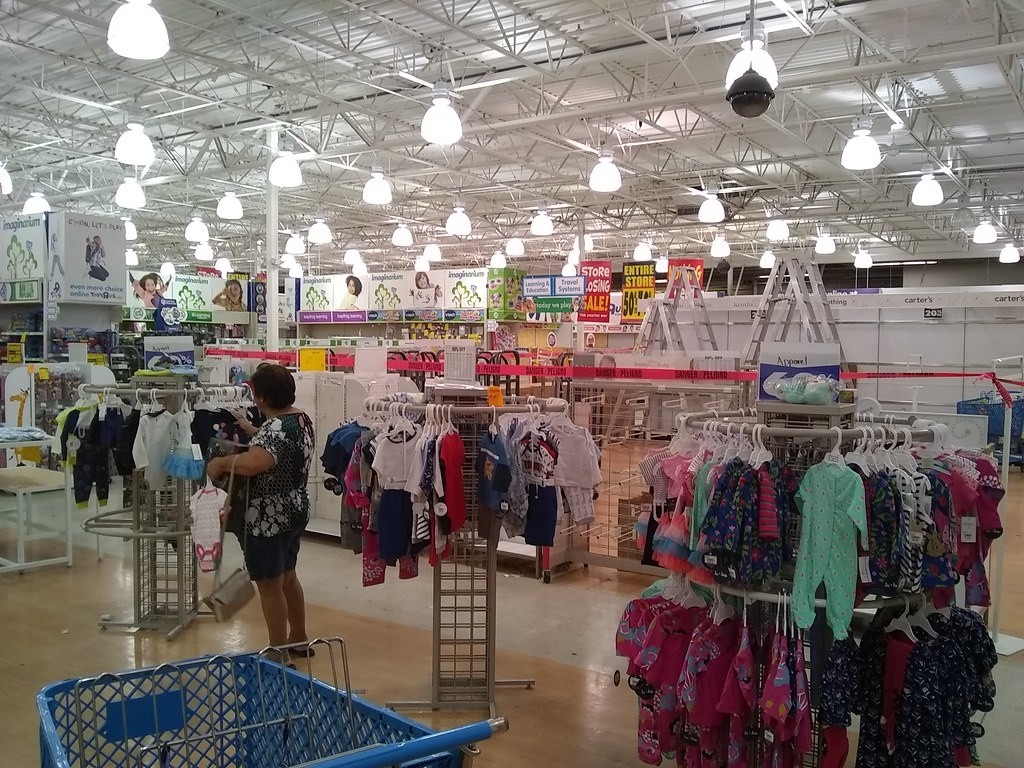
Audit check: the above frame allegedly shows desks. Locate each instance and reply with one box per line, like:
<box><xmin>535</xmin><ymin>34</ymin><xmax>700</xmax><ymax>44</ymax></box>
<box><xmin>0</xmin><ymin>466</ymin><xmax>104</xmax><ymax>574</ymax></box>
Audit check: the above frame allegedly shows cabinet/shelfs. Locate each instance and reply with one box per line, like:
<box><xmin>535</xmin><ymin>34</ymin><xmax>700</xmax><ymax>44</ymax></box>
<box><xmin>0</xmin><ymin>277</ymin><xmax>121</xmax><ymax>380</ymax></box>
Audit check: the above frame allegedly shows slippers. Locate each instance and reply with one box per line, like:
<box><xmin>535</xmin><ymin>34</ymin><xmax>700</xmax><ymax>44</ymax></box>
<box><xmin>287</xmin><ymin>663</ymin><xmax>296</xmax><ymax>671</ymax></box>
<box><xmin>288</xmin><ymin>647</ymin><xmax>315</xmax><ymax>658</ymax></box>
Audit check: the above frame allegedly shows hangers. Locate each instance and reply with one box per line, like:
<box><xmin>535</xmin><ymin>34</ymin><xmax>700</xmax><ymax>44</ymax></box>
<box><xmin>57</xmin><ymin>381</ymin><xmax>264</xmax><ymax>426</ymax></box>
<box><xmin>328</xmin><ymin>393</ymin><xmax>593</xmax><ymax>453</ymax></box>
<box><xmin>203</xmin><ymin>475</ymin><xmax>217</xmax><ymax>491</ymax></box>
<box><xmin>639</xmin><ymin>407</ymin><xmax>998</xmax><ymax>526</ymax></box>
<box><xmin>627</xmin><ymin>568</ymin><xmax>988</xmax><ymax>689</ymax></box>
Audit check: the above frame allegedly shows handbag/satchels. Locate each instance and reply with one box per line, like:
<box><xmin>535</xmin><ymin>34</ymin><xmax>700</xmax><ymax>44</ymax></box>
<box><xmin>209</xmin><ymin>573</ymin><xmax>256</xmax><ymax>623</ymax></box>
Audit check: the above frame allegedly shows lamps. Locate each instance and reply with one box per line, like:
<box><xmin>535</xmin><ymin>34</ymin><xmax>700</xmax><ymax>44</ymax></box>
<box><xmin>307</xmin><ymin>209</ymin><xmax>333</xmax><ymax>243</ymax></box>
<box><xmin>0</xmin><ymin>161</ymin><xmax>13</xmax><ymax>194</ymax></box>
<box><xmin>115</xmin><ymin>177</ymin><xmax>146</xmax><ymax>209</ymax></box>
<box><xmin>23</xmin><ymin>179</ymin><xmax>51</xmax><ymax>221</ymax></box>
<box><xmin>217</xmin><ymin>185</ymin><xmax>243</xmax><ymax>219</ymax></box>
<box><xmin>160</xmin><ymin>262</ymin><xmax>175</xmax><ymax>274</ymax></box>
<box><xmin>194</xmin><ymin>242</ymin><xmax>213</xmax><ymax>260</ymax></box>
<box><xmin>280</xmin><ymin>254</ymin><xmax>296</xmax><ymax>268</ymax></box>
<box><xmin>269</xmin><ymin>138</ymin><xmax>303</xmax><ymax>187</ymax></box>
<box><xmin>911</xmin><ymin>154</ymin><xmax>944</xmax><ymax>206</ymax></box>
<box><xmin>998</xmin><ymin>241</ymin><xmax>1020</xmax><ymax>263</ymax></box>
<box><xmin>107</xmin><ymin>0</ymin><xmax>170</xmax><ymax>60</ymax></box>
<box><xmin>972</xmin><ymin>209</ymin><xmax>997</xmax><ymax>243</ymax></box>
<box><xmin>185</xmin><ymin>207</ymin><xmax>209</xmax><ymax>242</ymax></box>
<box><xmin>344</xmin><ymin>21</ymin><xmax>882</xmax><ymax>277</ymax></box>
<box><xmin>114</xmin><ymin>112</ymin><xmax>155</xmax><ymax>165</ymax></box>
<box><xmin>289</xmin><ymin>263</ymin><xmax>303</xmax><ymax>278</ymax></box>
<box><xmin>285</xmin><ymin>225</ymin><xmax>306</xmax><ymax>254</ymax></box>
<box><xmin>126</xmin><ymin>247</ymin><xmax>138</xmax><ymax>265</ymax></box>
<box><xmin>120</xmin><ymin>214</ymin><xmax>137</xmax><ymax>240</ymax></box>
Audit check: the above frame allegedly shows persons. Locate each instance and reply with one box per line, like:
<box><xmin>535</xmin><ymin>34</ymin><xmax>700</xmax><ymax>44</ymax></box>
<box><xmin>50</xmin><ymin>234</ymin><xmax>64</xmax><ymax>276</ymax></box>
<box><xmin>86</xmin><ymin>235</ymin><xmax>109</xmax><ymax>281</ymax></box>
<box><xmin>409</xmin><ymin>272</ymin><xmax>443</xmax><ymax>309</ymax></box>
<box><xmin>206</xmin><ymin>365</ymin><xmax>316</xmax><ymax>670</ymax></box>
<box><xmin>50</xmin><ymin>282</ymin><xmax>62</xmax><ymax>299</ymax></box>
<box><xmin>212</xmin><ymin>279</ymin><xmax>246</xmax><ymax>311</ymax></box>
<box><xmin>129</xmin><ymin>272</ymin><xmax>172</xmax><ymax>309</ymax></box>
<box><xmin>342</xmin><ymin>275</ymin><xmax>365</xmax><ymax>310</ymax></box>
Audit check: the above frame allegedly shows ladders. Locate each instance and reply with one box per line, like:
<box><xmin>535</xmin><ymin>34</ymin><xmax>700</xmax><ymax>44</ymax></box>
<box><xmin>656</xmin><ymin>266</ymin><xmax>719</xmax><ymax>350</ymax></box>
<box><xmin>741</xmin><ymin>253</ymin><xmax>855</xmax><ymax>391</ymax></box>
<box><xmin>601</xmin><ymin>298</ymin><xmax>704</xmax><ymax>451</ymax></box>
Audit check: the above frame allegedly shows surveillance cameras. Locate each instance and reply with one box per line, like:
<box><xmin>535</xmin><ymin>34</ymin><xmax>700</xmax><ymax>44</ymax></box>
<box><xmin>718</xmin><ymin>260</ymin><xmax>731</xmax><ymax>273</ymax></box>
<box><xmin>726</xmin><ymin>69</ymin><xmax>775</xmax><ymax>118</ymax></box>
